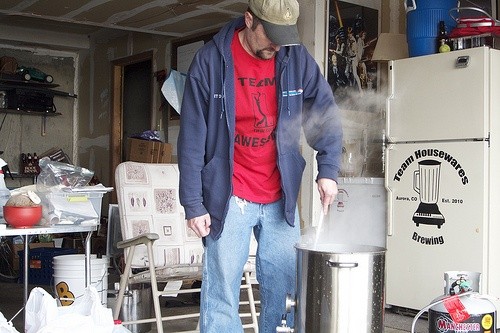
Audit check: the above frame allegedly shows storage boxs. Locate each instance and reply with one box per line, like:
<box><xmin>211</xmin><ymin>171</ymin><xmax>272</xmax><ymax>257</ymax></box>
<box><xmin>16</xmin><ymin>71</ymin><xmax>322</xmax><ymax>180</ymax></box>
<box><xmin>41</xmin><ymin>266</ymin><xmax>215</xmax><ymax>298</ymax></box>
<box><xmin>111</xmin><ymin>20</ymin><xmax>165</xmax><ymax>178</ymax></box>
<box><xmin>126</xmin><ymin>137</ymin><xmax>172</xmax><ymax>163</ymax></box>
<box><xmin>29</xmin><ymin>192</ymin><xmax>107</xmax><ymax>227</ymax></box>
<box><xmin>17</xmin><ymin>246</ymin><xmax>77</xmax><ymax>286</ymax></box>
<box><xmin>8</xmin><ymin>242</ymin><xmax>54</xmax><ymax>271</ymax></box>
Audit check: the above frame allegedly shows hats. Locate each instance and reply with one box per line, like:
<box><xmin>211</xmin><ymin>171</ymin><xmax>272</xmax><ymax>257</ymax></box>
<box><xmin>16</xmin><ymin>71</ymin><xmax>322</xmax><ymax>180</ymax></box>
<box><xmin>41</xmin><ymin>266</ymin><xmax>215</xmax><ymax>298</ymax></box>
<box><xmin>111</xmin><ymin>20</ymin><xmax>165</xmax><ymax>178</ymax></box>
<box><xmin>247</xmin><ymin>0</ymin><xmax>301</xmax><ymax>47</ymax></box>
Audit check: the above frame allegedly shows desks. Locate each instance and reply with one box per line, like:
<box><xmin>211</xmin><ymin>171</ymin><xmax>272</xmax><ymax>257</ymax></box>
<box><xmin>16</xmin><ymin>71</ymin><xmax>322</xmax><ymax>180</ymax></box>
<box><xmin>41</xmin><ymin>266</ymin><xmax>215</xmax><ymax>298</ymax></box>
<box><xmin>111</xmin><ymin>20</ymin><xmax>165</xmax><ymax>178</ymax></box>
<box><xmin>0</xmin><ymin>222</ymin><xmax>97</xmax><ymax>333</ymax></box>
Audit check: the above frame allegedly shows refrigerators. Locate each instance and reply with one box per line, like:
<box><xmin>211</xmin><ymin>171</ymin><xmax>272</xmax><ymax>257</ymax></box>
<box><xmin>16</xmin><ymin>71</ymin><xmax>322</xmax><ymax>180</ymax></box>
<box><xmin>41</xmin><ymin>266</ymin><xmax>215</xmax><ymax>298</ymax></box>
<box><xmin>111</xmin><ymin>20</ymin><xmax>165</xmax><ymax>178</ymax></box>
<box><xmin>380</xmin><ymin>45</ymin><xmax>500</xmax><ymax>313</ymax></box>
<box><xmin>319</xmin><ymin>177</ymin><xmax>386</xmax><ymax>248</ymax></box>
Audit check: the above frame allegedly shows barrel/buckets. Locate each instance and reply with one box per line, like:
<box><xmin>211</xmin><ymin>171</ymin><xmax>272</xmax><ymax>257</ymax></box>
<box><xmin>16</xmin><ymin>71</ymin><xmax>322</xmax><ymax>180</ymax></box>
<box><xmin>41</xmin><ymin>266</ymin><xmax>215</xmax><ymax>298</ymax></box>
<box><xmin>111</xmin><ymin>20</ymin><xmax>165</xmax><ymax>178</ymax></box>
<box><xmin>404</xmin><ymin>0</ymin><xmax>460</xmax><ymax>57</ymax></box>
<box><xmin>428</xmin><ymin>306</ymin><xmax>497</xmax><ymax>333</ymax></box>
<box><xmin>51</xmin><ymin>254</ymin><xmax>109</xmax><ymax>308</ymax></box>
<box><xmin>107</xmin><ymin>282</ymin><xmax>151</xmax><ymax>333</ymax></box>
<box><xmin>293</xmin><ymin>243</ymin><xmax>387</xmax><ymax>333</ymax></box>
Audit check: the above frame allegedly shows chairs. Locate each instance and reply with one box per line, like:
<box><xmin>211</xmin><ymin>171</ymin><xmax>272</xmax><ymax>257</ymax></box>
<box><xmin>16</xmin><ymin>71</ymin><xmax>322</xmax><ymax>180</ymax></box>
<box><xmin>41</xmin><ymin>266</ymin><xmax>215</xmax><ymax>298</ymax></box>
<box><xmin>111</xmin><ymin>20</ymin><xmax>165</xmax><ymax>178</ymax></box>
<box><xmin>115</xmin><ymin>161</ymin><xmax>258</xmax><ymax>333</ymax></box>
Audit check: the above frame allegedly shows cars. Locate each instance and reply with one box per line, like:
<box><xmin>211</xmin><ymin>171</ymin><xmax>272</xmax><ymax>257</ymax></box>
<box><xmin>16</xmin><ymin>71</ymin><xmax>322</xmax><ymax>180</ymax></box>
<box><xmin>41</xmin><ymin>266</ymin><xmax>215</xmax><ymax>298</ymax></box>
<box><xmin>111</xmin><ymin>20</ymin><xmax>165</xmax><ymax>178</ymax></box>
<box><xmin>17</xmin><ymin>66</ymin><xmax>54</xmax><ymax>83</ymax></box>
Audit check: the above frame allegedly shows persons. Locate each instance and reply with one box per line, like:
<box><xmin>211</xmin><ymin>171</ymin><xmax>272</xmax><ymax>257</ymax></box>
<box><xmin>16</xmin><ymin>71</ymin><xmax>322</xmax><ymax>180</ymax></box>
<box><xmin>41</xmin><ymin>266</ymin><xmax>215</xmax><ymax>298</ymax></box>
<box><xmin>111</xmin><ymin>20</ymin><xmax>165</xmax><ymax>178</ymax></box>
<box><xmin>177</xmin><ymin>0</ymin><xmax>343</xmax><ymax>333</ymax></box>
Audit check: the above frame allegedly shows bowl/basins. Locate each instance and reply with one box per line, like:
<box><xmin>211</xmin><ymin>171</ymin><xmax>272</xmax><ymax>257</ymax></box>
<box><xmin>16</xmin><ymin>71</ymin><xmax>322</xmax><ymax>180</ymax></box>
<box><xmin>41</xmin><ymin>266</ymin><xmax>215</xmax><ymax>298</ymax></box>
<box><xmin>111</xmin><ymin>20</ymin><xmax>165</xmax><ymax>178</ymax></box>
<box><xmin>470</xmin><ymin>37</ymin><xmax>487</xmax><ymax>47</ymax></box>
<box><xmin>453</xmin><ymin>40</ymin><xmax>470</xmax><ymax>50</ymax></box>
<box><xmin>3</xmin><ymin>205</ymin><xmax>42</xmax><ymax>228</ymax></box>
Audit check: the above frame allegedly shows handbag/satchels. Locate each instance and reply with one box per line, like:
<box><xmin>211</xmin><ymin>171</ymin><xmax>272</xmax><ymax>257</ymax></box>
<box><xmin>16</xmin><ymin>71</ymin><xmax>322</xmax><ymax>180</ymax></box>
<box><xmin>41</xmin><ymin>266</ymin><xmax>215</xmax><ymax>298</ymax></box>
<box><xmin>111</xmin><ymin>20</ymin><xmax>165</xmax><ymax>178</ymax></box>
<box><xmin>24</xmin><ymin>285</ymin><xmax>115</xmax><ymax>333</ymax></box>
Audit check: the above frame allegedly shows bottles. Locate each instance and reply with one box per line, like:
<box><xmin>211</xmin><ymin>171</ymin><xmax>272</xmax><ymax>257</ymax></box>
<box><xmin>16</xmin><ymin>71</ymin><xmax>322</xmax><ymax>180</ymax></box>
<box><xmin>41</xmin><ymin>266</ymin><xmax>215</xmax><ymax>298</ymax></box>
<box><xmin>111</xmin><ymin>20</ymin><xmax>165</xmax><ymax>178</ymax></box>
<box><xmin>22</xmin><ymin>153</ymin><xmax>40</xmax><ymax>174</ymax></box>
<box><xmin>437</xmin><ymin>21</ymin><xmax>450</xmax><ymax>53</ymax></box>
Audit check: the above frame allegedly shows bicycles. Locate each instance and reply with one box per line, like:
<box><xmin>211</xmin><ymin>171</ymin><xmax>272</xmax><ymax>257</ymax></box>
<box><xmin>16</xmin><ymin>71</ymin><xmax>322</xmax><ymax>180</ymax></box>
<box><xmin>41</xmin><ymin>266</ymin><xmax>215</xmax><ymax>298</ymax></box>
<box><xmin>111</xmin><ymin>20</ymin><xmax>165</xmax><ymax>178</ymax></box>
<box><xmin>0</xmin><ymin>237</ymin><xmax>23</xmax><ymax>282</ymax></box>
